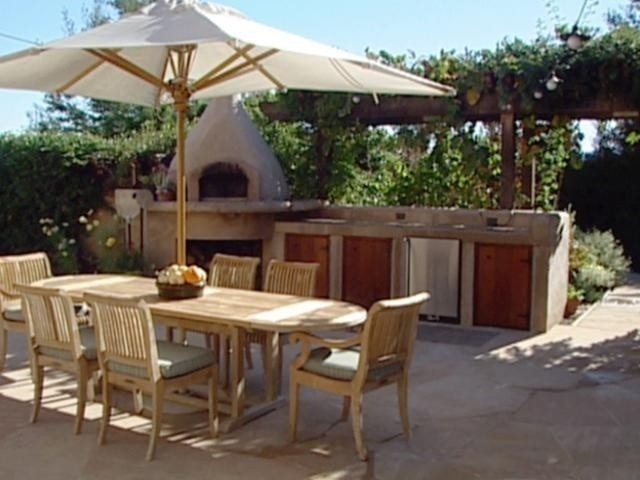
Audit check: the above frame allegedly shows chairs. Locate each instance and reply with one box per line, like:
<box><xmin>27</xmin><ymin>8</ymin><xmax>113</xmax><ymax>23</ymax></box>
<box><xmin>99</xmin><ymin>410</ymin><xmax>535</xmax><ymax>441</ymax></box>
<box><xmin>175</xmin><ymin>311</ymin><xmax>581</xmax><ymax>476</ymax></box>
<box><xmin>12</xmin><ymin>280</ymin><xmax>100</xmax><ymax>434</ymax></box>
<box><xmin>289</xmin><ymin>291</ymin><xmax>431</xmax><ymax>461</ymax></box>
<box><xmin>0</xmin><ymin>252</ymin><xmax>52</xmax><ymax>365</ymax></box>
<box><xmin>83</xmin><ymin>288</ymin><xmax>219</xmax><ymax>458</ymax></box>
<box><xmin>166</xmin><ymin>253</ymin><xmax>260</xmax><ymax>370</ymax></box>
<box><xmin>239</xmin><ymin>258</ymin><xmax>321</xmax><ymax>396</ymax></box>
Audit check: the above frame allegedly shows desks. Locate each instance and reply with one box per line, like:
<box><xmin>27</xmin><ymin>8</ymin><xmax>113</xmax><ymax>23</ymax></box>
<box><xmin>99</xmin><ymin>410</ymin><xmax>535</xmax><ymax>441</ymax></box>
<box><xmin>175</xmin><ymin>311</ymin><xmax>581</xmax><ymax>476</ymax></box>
<box><xmin>28</xmin><ymin>273</ymin><xmax>367</xmax><ymax>435</ymax></box>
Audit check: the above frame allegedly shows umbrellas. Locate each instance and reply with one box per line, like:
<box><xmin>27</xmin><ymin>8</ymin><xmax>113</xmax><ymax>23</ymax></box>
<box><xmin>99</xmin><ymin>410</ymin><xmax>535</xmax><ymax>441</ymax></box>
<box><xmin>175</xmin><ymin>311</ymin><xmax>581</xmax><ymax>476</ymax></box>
<box><xmin>0</xmin><ymin>0</ymin><xmax>457</xmax><ymax>396</ymax></box>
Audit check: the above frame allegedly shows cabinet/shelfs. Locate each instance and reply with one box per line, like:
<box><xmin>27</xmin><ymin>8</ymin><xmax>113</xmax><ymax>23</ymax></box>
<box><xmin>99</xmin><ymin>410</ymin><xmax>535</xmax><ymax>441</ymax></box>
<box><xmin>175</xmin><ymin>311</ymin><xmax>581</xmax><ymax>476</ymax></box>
<box><xmin>403</xmin><ymin>235</ymin><xmax>460</xmax><ymax>326</ymax></box>
<box><xmin>342</xmin><ymin>235</ymin><xmax>392</xmax><ymax>311</ymax></box>
<box><xmin>284</xmin><ymin>232</ymin><xmax>331</xmax><ymax>298</ymax></box>
<box><xmin>473</xmin><ymin>241</ymin><xmax>533</xmax><ymax>331</ymax></box>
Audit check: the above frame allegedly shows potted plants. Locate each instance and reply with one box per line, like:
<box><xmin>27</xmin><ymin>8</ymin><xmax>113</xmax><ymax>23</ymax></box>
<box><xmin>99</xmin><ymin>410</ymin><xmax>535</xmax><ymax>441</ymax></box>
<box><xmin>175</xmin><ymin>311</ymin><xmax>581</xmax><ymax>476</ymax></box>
<box><xmin>565</xmin><ymin>285</ymin><xmax>584</xmax><ymax>315</ymax></box>
